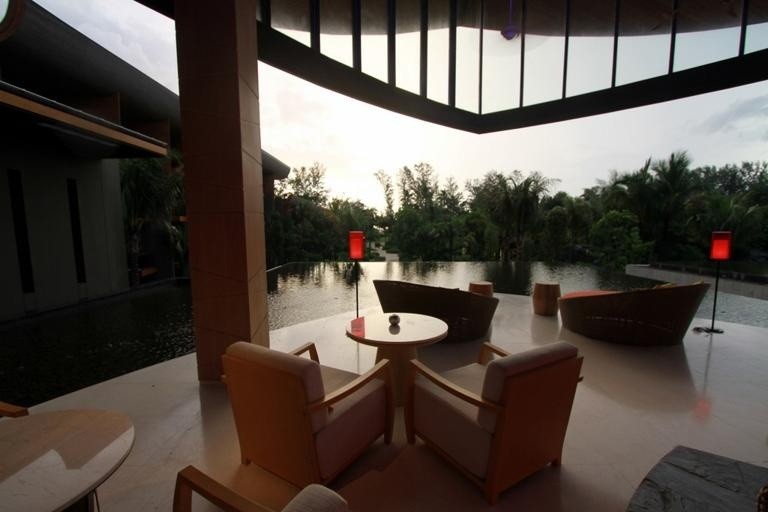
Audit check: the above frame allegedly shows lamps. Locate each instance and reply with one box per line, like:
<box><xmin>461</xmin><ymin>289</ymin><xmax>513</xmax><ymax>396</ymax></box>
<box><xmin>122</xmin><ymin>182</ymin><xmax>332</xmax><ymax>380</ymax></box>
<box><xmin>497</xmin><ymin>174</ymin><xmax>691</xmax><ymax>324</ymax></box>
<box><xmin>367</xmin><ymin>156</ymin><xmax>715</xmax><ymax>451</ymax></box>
<box><xmin>693</xmin><ymin>231</ymin><xmax>731</xmax><ymax>333</ymax></box>
<box><xmin>502</xmin><ymin>0</ymin><xmax>518</xmax><ymax>40</ymax></box>
<box><xmin>349</xmin><ymin>230</ymin><xmax>364</xmax><ymax>318</ymax></box>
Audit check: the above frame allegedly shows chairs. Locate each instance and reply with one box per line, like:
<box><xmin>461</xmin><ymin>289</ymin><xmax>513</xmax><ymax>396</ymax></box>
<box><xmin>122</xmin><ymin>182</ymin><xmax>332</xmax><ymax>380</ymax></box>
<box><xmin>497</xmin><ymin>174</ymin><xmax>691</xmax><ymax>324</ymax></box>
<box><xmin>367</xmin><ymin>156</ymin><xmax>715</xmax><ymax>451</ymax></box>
<box><xmin>174</xmin><ymin>466</ymin><xmax>351</xmax><ymax>512</ymax></box>
<box><xmin>557</xmin><ymin>282</ymin><xmax>711</xmax><ymax>346</ymax></box>
<box><xmin>372</xmin><ymin>280</ymin><xmax>499</xmax><ymax>342</ymax></box>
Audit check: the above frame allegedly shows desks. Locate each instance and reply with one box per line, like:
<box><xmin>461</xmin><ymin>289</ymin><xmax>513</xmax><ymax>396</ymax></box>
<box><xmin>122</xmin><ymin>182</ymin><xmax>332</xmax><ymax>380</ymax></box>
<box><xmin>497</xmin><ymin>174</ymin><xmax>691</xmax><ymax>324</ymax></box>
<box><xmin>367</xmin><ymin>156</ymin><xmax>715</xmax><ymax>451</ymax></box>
<box><xmin>0</xmin><ymin>408</ymin><xmax>134</xmax><ymax>512</ymax></box>
<box><xmin>346</xmin><ymin>312</ymin><xmax>448</xmax><ymax>406</ymax></box>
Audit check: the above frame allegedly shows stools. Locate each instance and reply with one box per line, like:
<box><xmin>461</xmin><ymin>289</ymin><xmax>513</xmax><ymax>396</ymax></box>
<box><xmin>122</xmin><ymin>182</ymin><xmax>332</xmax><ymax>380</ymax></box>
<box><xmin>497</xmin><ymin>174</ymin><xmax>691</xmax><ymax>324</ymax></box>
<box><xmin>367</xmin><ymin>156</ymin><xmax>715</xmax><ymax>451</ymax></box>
<box><xmin>533</xmin><ymin>282</ymin><xmax>561</xmax><ymax>316</ymax></box>
<box><xmin>469</xmin><ymin>281</ymin><xmax>493</xmax><ymax>297</ymax></box>
